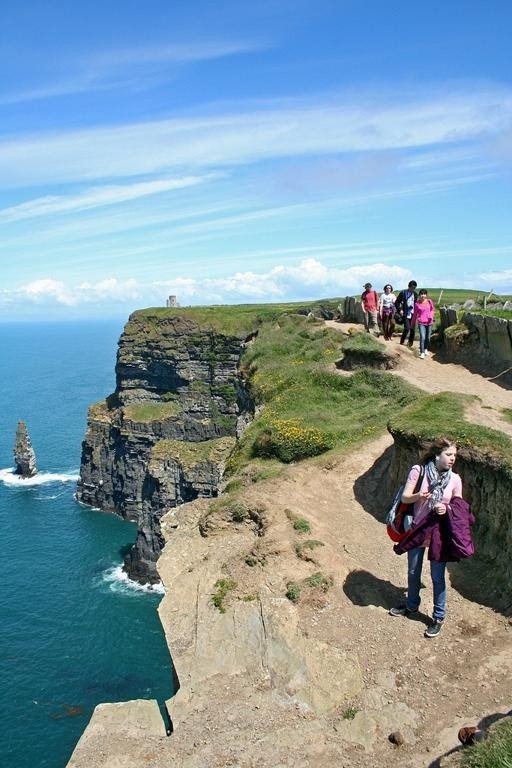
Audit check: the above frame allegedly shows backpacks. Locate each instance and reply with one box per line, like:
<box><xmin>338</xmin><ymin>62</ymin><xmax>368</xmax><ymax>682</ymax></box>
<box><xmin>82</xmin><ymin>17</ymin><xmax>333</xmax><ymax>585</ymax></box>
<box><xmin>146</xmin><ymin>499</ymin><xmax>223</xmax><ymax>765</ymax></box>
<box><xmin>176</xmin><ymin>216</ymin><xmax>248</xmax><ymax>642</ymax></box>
<box><xmin>385</xmin><ymin>464</ymin><xmax>425</xmax><ymax>543</ymax></box>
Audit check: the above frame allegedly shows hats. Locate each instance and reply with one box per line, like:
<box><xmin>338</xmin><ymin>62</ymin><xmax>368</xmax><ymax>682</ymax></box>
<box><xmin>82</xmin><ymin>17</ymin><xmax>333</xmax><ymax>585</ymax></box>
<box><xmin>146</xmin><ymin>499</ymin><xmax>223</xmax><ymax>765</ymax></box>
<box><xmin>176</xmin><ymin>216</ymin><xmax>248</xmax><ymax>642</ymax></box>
<box><xmin>363</xmin><ymin>283</ymin><xmax>372</xmax><ymax>288</ymax></box>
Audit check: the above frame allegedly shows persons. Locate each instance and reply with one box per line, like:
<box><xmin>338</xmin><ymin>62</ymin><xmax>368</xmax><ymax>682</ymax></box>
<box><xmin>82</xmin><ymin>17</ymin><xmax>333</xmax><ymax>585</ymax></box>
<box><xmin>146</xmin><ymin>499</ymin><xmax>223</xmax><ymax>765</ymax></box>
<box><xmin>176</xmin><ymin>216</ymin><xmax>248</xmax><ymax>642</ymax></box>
<box><xmin>360</xmin><ymin>283</ymin><xmax>380</xmax><ymax>337</ymax></box>
<box><xmin>379</xmin><ymin>285</ymin><xmax>398</xmax><ymax>341</ymax></box>
<box><xmin>394</xmin><ymin>281</ymin><xmax>418</xmax><ymax>347</ymax></box>
<box><xmin>410</xmin><ymin>289</ymin><xmax>435</xmax><ymax>360</ymax></box>
<box><xmin>389</xmin><ymin>437</ymin><xmax>463</xmax><ymax>637</ymax></box>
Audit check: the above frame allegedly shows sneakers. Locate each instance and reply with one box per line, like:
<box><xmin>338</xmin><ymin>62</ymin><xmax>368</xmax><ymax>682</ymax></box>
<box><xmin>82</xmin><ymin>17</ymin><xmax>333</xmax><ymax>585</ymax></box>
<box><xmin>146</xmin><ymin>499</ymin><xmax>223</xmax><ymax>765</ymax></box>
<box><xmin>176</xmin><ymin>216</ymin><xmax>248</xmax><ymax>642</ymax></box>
<box><xmin>389</xmin><ymin>603</ymin><xmax>419</xmax><ymax>618</ymax></box>
<box><xmin>424</xmin><ymin>618</ymin><xmax>446</xmax><ymax>637</ymax></box>
<box><xmin>420</xmin><ymin>352</ymin><xmax>425</xmax><ymax>359</ymax></box>
<box><xmin>425</xmin><ymin>349</ymin><xmax>430</xmax><ymax>356</ymax></box>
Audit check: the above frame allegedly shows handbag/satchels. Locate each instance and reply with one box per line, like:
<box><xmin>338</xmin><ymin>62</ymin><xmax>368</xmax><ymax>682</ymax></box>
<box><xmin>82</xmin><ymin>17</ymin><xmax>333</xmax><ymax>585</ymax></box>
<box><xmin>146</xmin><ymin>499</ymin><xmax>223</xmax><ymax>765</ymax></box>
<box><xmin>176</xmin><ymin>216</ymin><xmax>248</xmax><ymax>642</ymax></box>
<box><xmin>393</xmin><ymin>311</ymin><xmax>405</xmax><ymax>325</ymax></box>
